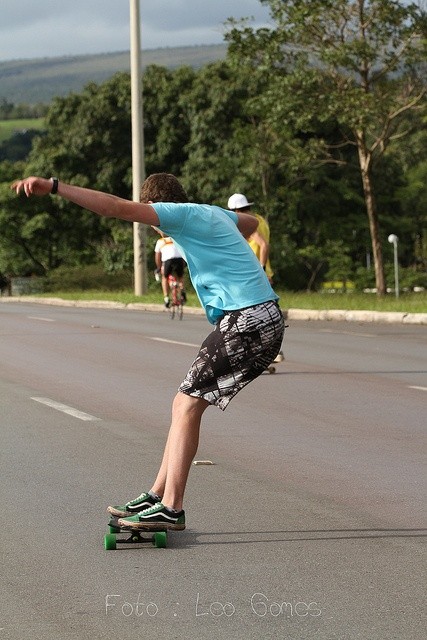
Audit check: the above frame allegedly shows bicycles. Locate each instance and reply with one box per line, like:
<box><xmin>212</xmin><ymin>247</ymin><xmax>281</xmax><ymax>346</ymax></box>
<box><xmin>154</xmin><ymin>268</ymin><xmax>188</xmax><ymax>321</ymax></box>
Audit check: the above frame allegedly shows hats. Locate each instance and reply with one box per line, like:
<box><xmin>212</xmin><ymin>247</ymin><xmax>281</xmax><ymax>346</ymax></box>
<box><xmin>227</xmin><ymin>193</ymin><xmax>254</xmax><ymax>209</ymax></box>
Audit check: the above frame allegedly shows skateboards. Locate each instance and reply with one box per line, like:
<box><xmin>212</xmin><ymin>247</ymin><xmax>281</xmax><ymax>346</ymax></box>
<box><xmin>102</xmin><ymin>503</ymin><xmax>168</xmax><ymax>551</ymax></box>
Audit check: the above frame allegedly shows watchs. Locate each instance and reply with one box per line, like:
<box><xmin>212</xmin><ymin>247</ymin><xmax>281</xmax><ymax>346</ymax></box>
<box><xmin>50</xmin><ymin>177</ymin><xmax>59</xmax><ymax>195</ymax></box>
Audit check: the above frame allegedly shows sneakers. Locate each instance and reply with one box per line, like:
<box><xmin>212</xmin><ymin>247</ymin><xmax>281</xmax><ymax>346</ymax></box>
<box><xmin>117</xmin><ymin>502</ymin><xmax>186</xmax><ymax>531</ymax></box>
<box><xmin>106</xmin><ymin>492</ymin><xmax>163</xmax><ymax>518</ymax></box>
<box><xmin>181</xmin><ymin>292</ymin><xmax>186</xmax><ymax>302</ymax></box>
<box><xmin>163</xmin><ymin>297</ymin><xmax>170</xmax><ymax>308</ymax></box>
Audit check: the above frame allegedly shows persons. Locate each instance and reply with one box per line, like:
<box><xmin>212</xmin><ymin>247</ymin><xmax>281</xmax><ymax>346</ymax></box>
<box><xmin>227</xmin><ymin>194</ymin><xmax>273</xmax><ymax>288</ymax></box>
<box><xmin>154</xmin><ymin>233</ymin><xmax>186</xmax><ymax>308</ymax></box>
<box><xmin>8</xmin><ymin>174</ymin><xmax>285</xmax><ymax>530</ymax></box>
